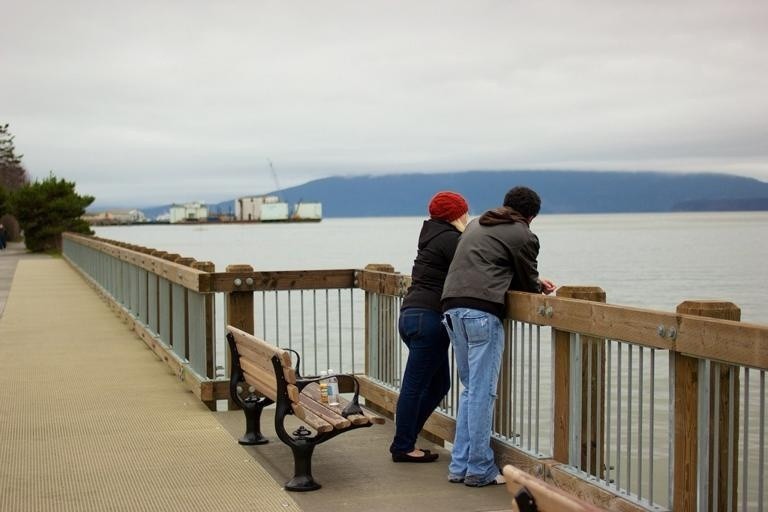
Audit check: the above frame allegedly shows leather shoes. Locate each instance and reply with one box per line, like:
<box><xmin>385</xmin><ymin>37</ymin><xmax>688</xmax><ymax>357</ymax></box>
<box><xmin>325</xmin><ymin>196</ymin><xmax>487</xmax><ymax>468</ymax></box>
<box><xmin>390</xmin><ymin>442</ymin><xmax>439</xmax><ymax>463</ymax></box>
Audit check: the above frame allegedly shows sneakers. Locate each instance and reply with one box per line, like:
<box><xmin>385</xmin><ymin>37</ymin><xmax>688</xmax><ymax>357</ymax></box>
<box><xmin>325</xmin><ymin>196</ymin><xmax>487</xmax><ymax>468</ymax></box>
<box><xmin>448</xmin><ymin>469</ymin><xmax>505</xmax><ymax>487</ymax></box>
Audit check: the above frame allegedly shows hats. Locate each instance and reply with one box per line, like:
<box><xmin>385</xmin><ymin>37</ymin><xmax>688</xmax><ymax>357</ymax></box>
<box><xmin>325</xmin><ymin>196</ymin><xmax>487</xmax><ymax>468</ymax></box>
<box><xmin>428</xmin><ymin>192</ymin><xmax>468</xmax><ymax>223</ymax></box>
<box><xmin>504</xmin><ymin>187</ymin><xmax>541</xmax><ymax>217</ymax></box>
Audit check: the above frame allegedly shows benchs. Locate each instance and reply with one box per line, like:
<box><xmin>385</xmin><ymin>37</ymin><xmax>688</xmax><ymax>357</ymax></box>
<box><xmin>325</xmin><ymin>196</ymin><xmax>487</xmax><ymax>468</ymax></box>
<box><xmin>224</xmin><ymin>325</ymin><xmax>387</xmax><ymax>492</ymax></box>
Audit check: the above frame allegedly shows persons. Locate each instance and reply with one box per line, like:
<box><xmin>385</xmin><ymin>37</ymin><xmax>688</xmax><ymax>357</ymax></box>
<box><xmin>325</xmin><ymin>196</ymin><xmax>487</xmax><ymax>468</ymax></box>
<box><xmin>440</xmin><ymin>185</ymin><xmax>558</xmax><ymax>488</ymax></box>
<box><xmin>388</xmin><ymin>191</ymin><xmax>470</xmax><ymax>463</ymax></box>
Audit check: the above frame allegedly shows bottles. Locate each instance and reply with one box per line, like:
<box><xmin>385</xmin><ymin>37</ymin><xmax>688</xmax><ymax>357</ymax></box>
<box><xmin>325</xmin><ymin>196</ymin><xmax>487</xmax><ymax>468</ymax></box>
<box><xmin>320</xmin><ymin>369</ymin><xmax>340</xmax><ymax>406</ymax></box>
